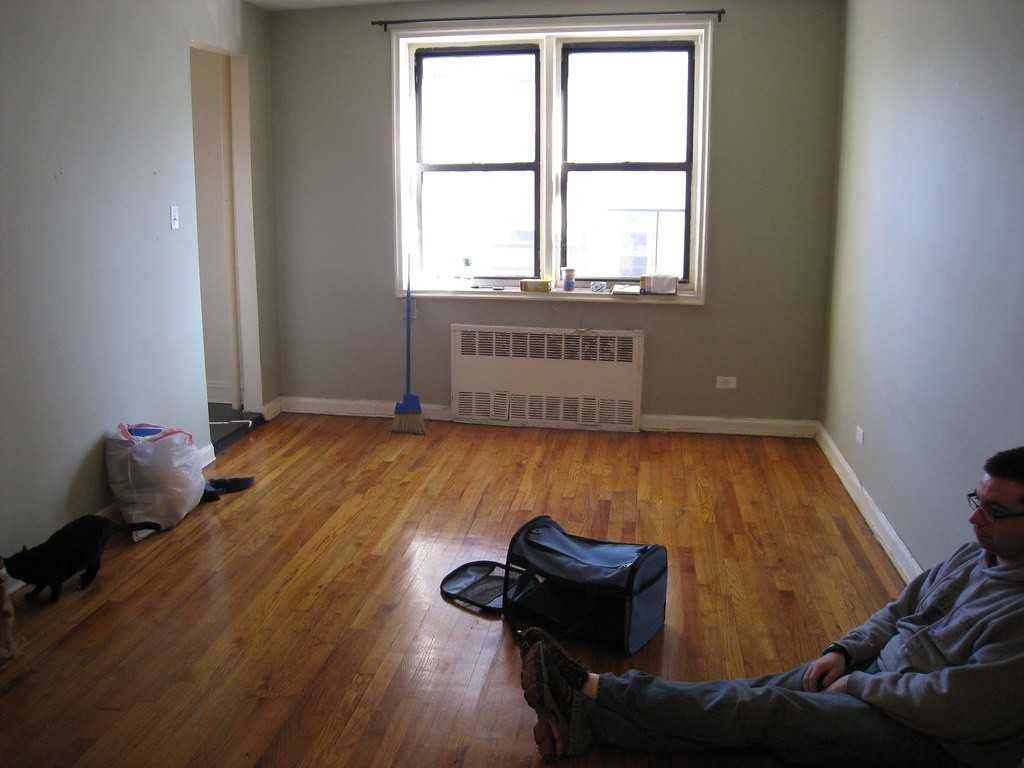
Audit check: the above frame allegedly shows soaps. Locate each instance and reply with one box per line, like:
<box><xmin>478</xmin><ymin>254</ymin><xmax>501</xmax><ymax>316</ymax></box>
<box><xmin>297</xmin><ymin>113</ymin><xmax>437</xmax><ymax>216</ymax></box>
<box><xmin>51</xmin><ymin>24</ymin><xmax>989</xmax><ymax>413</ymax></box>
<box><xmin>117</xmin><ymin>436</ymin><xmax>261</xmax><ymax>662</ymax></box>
<box><xmin>520</xmin><ymin>278</ymin><xmax>552</xmax><ymax>291</ymax></box>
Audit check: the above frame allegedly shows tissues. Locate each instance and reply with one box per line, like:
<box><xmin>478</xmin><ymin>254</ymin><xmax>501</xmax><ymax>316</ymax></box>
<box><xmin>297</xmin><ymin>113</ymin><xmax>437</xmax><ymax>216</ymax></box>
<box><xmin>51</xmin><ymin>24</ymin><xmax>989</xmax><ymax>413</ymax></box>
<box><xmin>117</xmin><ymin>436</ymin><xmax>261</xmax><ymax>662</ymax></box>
<box><xmin>640</xmin><ymin>271</ymin><xmax>680</xmax><ymax>296</ymax></box>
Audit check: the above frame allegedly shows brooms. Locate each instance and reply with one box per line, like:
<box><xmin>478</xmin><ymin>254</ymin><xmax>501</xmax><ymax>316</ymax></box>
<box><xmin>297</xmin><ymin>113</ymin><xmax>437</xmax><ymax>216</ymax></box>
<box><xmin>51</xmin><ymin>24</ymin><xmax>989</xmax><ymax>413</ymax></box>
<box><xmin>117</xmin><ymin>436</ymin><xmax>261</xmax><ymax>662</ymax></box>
<box><xmin>387</xmin><ymin>250</ymin><xmax>427</xmax><ymax>436</ymax></box>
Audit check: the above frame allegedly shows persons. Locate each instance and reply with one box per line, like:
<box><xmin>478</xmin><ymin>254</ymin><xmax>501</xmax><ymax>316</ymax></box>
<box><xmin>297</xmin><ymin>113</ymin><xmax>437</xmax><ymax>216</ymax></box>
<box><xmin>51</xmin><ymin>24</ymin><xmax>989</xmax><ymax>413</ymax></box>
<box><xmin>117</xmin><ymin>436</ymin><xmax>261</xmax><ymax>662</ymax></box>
<box><xmin>520</xmin><ymin>445</ymin><xmax>1024</xmax><ymax>768</ymax></box>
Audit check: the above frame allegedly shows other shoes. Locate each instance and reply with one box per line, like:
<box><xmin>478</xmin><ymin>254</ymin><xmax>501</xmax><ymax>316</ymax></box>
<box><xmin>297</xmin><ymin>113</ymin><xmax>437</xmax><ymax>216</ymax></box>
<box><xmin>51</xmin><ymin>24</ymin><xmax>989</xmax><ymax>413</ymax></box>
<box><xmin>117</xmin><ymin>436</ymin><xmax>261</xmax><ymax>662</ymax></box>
<box><xmin>520</xmin><ymin>625</ymin><xmax>595</xmax><ymax>765</ymax></box>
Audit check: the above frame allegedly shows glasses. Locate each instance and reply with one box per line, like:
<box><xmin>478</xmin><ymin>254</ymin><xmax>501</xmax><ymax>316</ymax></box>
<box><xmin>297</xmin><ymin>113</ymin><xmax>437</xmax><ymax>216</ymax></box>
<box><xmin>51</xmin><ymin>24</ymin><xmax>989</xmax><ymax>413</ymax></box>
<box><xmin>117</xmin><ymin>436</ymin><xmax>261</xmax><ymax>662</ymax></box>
<box><xmin>966</xmin><ymin>492</ymin><xmax>1024</xmax><ymax>525</ymax></box>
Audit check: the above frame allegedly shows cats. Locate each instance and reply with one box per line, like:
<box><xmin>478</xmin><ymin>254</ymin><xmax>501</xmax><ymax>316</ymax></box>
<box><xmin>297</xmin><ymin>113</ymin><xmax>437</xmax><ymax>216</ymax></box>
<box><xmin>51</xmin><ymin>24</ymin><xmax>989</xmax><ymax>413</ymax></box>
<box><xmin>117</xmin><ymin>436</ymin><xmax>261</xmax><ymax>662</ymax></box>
<box><xmin>0</xmin><ymin>514</ymin><xmax>162</xmax><ymax>606</ymax></box>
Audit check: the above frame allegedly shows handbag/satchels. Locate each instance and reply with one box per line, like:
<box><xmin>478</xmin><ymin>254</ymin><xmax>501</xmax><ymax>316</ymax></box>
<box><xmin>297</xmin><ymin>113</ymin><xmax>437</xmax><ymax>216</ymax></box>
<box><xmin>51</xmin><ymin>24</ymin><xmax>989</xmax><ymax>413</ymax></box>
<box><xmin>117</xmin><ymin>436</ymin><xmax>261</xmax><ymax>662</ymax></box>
<box><xmin>103</xmin><ymin>419</ymin><xmax>205</xmax><ymax>541</ymax></box>
<box><xmin>438</xmin><ymin>513</ymin><xmax>669</xmax><ymax>657</ymax></box>
